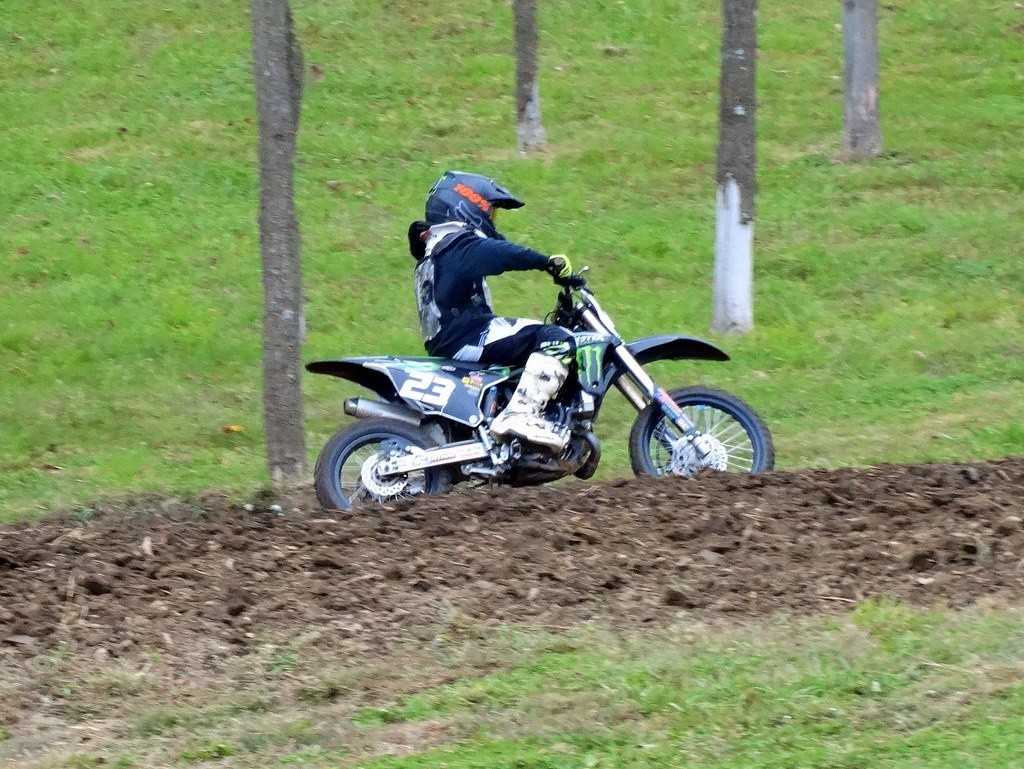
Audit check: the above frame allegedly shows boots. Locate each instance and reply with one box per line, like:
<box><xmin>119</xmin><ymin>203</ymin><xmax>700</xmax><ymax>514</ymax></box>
<box><xmin>489</xmin><ymin>352</ymin><xmax>566</xmax><ymax>454</ymax></box>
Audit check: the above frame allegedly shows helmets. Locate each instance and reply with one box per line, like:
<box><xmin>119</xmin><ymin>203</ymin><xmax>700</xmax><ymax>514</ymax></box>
<box><xmin>426</xmin><ymin>171</ymin><xmax>526</xmax><ymax>243</ymax></box>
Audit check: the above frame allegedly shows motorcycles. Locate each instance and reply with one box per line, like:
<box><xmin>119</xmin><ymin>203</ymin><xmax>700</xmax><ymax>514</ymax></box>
<box><xmin>302</xmin><ymin>257</ymin><xmax>776</xmax><ymax>514</ymax></box>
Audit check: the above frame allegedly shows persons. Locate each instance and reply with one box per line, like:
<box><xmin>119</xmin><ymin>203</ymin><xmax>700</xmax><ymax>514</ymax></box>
<box><xmin>407</xmin><ymin>171</ymin><xmax>577</xmax><ymax>451</ymax></box>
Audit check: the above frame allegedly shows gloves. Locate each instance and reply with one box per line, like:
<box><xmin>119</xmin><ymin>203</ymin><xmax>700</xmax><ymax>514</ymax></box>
<box><xmin>547</xmin><ymin>253</ymin><xmax>574</xmax><ymax>280</ymax></box>
